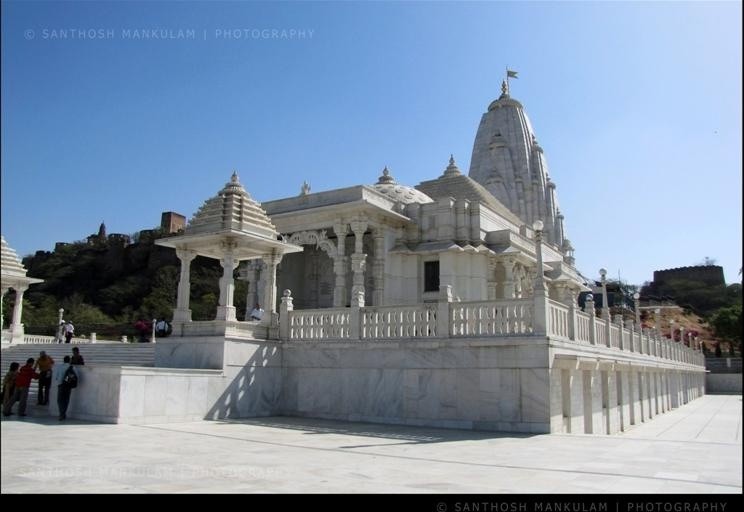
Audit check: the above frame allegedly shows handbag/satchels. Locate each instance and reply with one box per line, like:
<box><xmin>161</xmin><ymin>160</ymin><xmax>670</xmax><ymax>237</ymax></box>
<box><xmin>0</xmin><ymin>386</ymin><xmax>5</xmax><ymax>404</ymax></box>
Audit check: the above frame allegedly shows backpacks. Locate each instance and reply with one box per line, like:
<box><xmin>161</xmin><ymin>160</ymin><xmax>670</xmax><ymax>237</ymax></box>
<box><xmin>62</xmin><ymin>365</ymin><xmax>78</xmax><ymax>388</ymax></box>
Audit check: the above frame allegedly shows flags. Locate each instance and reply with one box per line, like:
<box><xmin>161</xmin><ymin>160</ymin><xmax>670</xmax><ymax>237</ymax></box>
<box><xmin>507</xmin><ymin>71</ymin><xmax>518</xmax><ymax>80</ymax></box>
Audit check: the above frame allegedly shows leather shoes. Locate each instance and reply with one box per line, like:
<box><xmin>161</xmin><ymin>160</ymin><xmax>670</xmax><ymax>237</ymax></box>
<box><xmin>3</xmin><ymin>411</ymin><xmax>26</xmax><ymax>416</ymax></box>
<box><xmin>42</xmin><ymin>401</ymin><xmax>47</xmax><ymax>406</ymax></box>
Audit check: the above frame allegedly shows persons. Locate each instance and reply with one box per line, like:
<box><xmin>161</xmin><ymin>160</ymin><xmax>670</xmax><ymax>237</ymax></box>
<box><xmin>54</xmin><ymin>320</ymin><xmax>65</xmax><ymax>344</ymax></box>
<box><xmin>1</xmin><ymin>346</ymin><xmax>85</xmax><ymax>419</ymax></box>
<box><xmin>65</xmin><ymin>320</ymin><xmax>75</xmax><ymax>343</ymax></box>
<box><xmin>250</xmin><ymin>302</ymin><xmax>264</xmax><ymax>321</ymax></box>
<box><xmin>156</xmin><ymin>319</ymin><xmax>169</xmax><ymax>338</ymax></box>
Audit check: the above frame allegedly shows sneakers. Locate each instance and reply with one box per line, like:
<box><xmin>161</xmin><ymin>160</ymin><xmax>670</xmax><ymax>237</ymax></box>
<box><xmin>60</xmin><ymin>414</ymin><xmax>65</xmax><ymax>420</ymax></box>
